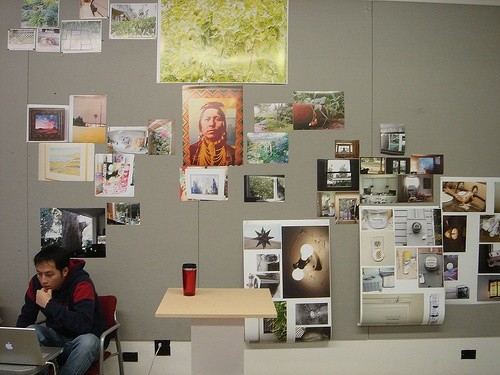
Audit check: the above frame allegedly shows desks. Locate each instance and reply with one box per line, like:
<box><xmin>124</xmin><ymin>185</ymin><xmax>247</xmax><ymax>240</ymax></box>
<box><xmin>154</xmin><ymin>288</ymin><xmax>277</xmax><ymax>375</ymax></box>
<box><xmin>0</xmin><ymin>347</ymin><xmax>64</xmax><ymax>375</ymax></box>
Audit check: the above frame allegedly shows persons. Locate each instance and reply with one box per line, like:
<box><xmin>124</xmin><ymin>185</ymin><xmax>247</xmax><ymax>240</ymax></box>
<box><xmin>190</xmin><ymin>102</ymin><xmax>235</xmax><ymax>166</ymax></box>
<box><xmin>16</xmin><ymin>245</ymin><xmax>110</xmax><ymax>375</ymax></box>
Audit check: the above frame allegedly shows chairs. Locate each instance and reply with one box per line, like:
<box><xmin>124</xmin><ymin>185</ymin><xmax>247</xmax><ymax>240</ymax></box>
<box><xmin>84</xmin><ymin>295</ymin><xmax>123</xmax><ymax>375</ymax></box>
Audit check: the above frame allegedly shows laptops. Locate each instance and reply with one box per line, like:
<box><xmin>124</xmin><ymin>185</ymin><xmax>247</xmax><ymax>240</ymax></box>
<box><xmin>0</xmin><ymin>326</ymin><xmax>64</xmax><ymax>365</ymax></box>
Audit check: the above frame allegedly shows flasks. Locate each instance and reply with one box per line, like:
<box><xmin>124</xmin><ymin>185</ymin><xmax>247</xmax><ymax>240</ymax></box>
<box><xmin>182</xmin><ymin>264</ymin><xmax>197</xmax><ymax>296</ymax></box>
